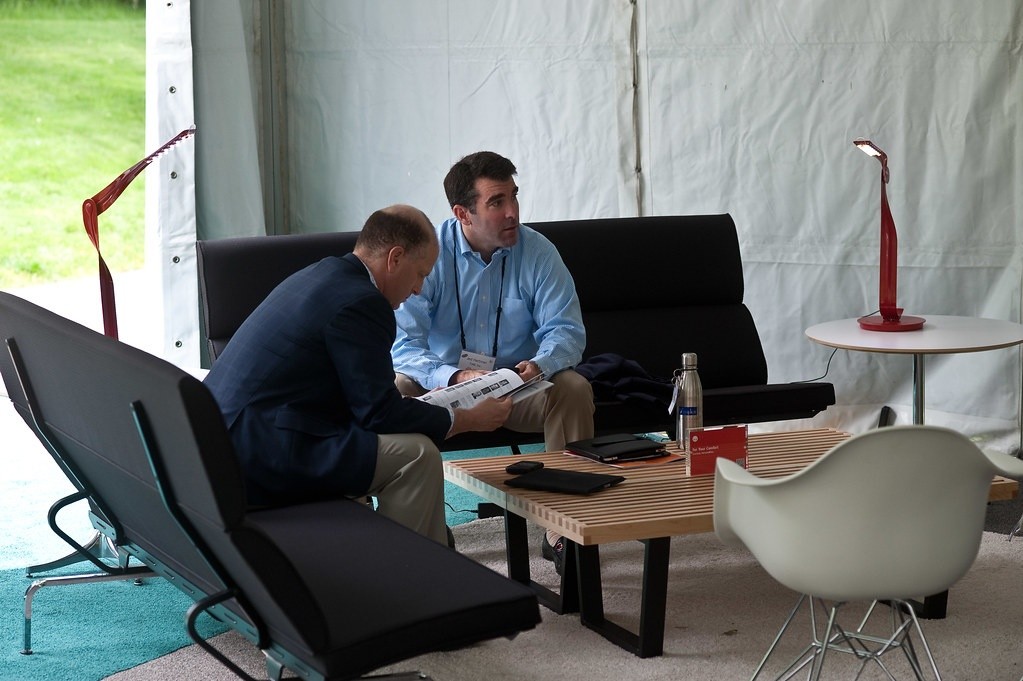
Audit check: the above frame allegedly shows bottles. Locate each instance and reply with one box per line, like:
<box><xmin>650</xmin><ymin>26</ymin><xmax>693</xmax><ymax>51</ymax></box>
<box><xmin>671</xmin><ymin>353</ymin><xmax>704</xmax><ymax>450</ymax></box>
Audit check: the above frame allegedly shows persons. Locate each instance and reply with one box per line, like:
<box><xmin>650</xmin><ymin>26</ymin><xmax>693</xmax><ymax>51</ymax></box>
<box><xmin>202</xmin><ymin>203</ymin><xmax>513</xmax><ymax>549</ymax></box>
<box><xmin>389</xmin><ymin>151</ymin><xmax>595</xmax><ymax>576</ymax></box>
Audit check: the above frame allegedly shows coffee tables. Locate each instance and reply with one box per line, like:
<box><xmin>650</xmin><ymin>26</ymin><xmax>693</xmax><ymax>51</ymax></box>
<box><xmin>443</xmin><ymin>426</ymin><xmax>1018</xmax><ymax>658</ymax></box>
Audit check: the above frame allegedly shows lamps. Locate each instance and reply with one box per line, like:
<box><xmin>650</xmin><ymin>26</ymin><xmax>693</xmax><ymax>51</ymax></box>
<box><xmin>27</xmin><ymin>130</ymin><xmax>193</xmax><ymax>585</ymax></box>
<box><xmin>852</xmin><ymin>136</ymin><xmax>927</xmax><ymax>332</ymax></box>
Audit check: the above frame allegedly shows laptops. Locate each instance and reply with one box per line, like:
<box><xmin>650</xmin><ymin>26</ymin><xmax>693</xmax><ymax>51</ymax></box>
<box><xmin>566</xmin><ymin>436</ymin><xmax>671</xmax><ymax>464</ymax></box>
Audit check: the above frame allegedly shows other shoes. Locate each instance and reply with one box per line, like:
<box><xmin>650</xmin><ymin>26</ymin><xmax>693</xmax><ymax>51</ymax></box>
<box><xmin>541</xmin><ymin>531</ymin><xmax>562</xmax><ymax>574</ymax></box>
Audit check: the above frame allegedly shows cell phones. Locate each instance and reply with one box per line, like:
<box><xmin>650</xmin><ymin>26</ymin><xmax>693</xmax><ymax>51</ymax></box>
<box><xmin>505</xmin><ymin>461</ymin><xmax>545</xmax><ymax>476</ymax></box>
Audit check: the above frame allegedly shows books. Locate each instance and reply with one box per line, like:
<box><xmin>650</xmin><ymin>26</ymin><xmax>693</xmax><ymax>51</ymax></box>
<box><xmin>415</xmin><ymin>368</ymin><xmax>554</xmax><ymax>410</ymax></box>
<box><xmin>564</xmin><ymin>451</ymin><xmax>686</xmax><ymax>469</ymax></box>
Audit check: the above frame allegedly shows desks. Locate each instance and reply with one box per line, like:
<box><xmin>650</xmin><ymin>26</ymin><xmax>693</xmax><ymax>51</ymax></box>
<box><xmin>805</xmin><ymin>315</ymin><xmax>1023</xmax><ymax>426</ymax></box>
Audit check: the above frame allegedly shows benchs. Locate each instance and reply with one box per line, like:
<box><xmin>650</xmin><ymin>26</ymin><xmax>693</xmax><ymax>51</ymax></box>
<box><xmin>196</xmin><ymin>212</ymin><xmax>837</xmax><ymax>453</ymax></box>
<box><xmin>0</xmin><ymin>291</ymin><xmax>541</xmax><ymax>681</ymax></box>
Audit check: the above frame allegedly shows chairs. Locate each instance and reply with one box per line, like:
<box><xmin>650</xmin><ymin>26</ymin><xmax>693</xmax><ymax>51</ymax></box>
<box><xmin>714</xmin><ymin>424</ymin><xmax>1023</xmax><ymax>681</ymax></box>
